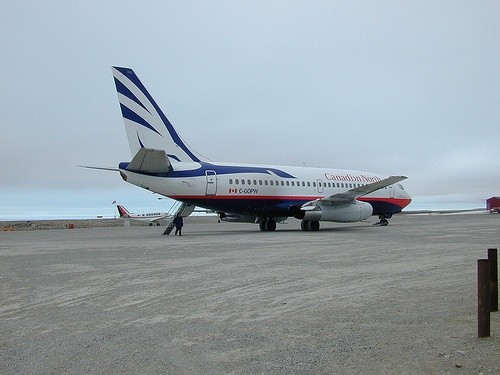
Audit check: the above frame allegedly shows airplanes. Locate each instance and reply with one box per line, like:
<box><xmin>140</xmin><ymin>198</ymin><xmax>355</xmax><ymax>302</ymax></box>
<box><xmin>74</xmin><ymin>66</ymin><xmax>413</xmax><ymax>233</ymax></box>
<box><xmin>116</xmin><ymin>204</ymin><xmax>173</xmax><ymax>226</ymax></box>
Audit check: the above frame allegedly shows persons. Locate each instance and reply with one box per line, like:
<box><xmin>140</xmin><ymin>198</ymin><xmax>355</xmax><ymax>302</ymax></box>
<box><xmin>173</xmin><ymin>213</ymin><xmax>183</xmax><ymax>236</ymax></box>
